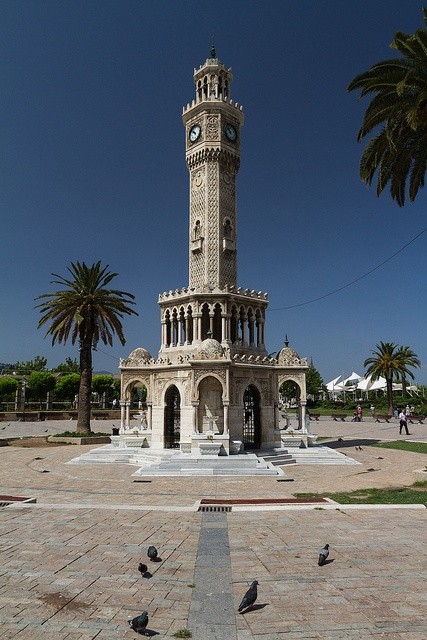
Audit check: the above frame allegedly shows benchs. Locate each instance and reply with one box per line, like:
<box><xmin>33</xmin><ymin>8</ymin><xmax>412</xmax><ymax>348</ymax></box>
<box><xmin>130</xmin><ymin>413</ymin><xmax>140</xmax><ymax>420</ymax></box>
<box><xmin>16</xmin><ymin>413</ymin><xmax>38</xmax><ymax>422</ymax></box>
<box><xmin>374</xmin><ymin>415</ymin><xmax>392</xmax><ymax>423</ymax></box>
<box><xmin>42</xmin><ymin>413</ymin><xmax>63</xmax><ymax>422</ymax></box>
<box><xmin>91</xmin><ymin>413</ymin><xmax>108</xmax><ymax>420</ymax></box>
<box><xmin>406</xmin><ymin>416</ymin><xmax>425</xmax><ymax>424</ymax></box>
<box><xmin>331</xmin><ymin>414</ymin><xmax>347</xmax><ymax>422</ymax></box>
<box><xmin>69</xmin><ymin>413</ymin><xmax>78</xmax><ymax>420</ymax></box>
<box><xmin>309</xmin><ymin>414</ymin><xmax>320</xmax><ymax>420</ymax></box>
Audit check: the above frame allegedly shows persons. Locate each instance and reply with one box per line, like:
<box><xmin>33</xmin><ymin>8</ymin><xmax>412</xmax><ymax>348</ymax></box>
<box><xmin>357</xmin><ymin>407</ymin><xmax>362</xmax><ymax>419</ymax></box>
<box><xmin>370</xmin><ymin>404</ymin><xmax>375</xmax><ymax>417</ymax></box>
<box><xmin>399</xmin><ymin>410</ymin><xmax>410</xmax><ymax>435</ymax></box>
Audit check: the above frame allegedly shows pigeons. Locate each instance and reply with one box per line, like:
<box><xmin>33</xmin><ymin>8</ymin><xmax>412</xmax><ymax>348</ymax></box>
<box><xmin>147</xmin><ymin>545</ymin><xmax>158</xmax><ymax>559</ymax></box>
<box><xmin>127</xmin><ymin>611</ymin><xmax>150</xmax><ymax>635</ymax></box>
<box><xmin>238</xmin><ymin>580</ymin><xmax>261</xmax><ymax>612</ymax></box>
<box><xmin>318</xmin><ymin>543</ymin><xmax>330</xmax><ymax>566</ymax></box>
<box><xmin>138</xmin><ymin>562</ymin><xmax>147</xmax><ymax>574</ymax></box>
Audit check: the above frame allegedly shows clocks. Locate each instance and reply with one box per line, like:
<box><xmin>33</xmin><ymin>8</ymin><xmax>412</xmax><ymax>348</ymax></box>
<box><xmin>189</xmin><ymin>125</ymin><xmax>200</xmax><ymax>141</ymax></box>
<box><xmin>224</xmin><ymin>124</ymin><xmax>237</xmax><ymax>141</ymax></box>
